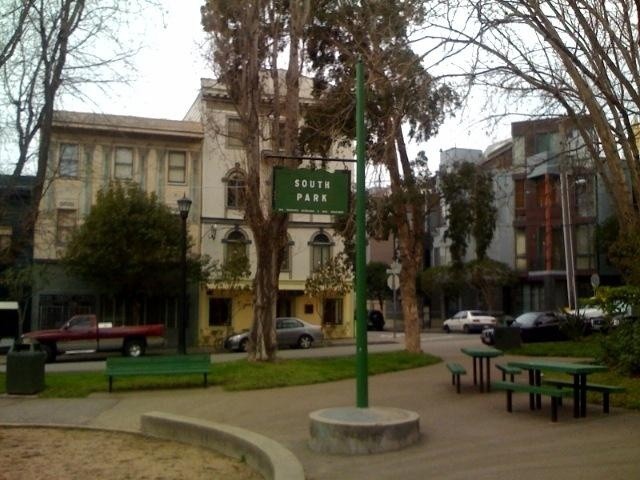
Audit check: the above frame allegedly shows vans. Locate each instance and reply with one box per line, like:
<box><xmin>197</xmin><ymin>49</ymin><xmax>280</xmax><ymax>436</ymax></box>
<box><xmin>0</xmin><ymin>298</ymin><xmax>24</xmax><ymax>365</ymax></box>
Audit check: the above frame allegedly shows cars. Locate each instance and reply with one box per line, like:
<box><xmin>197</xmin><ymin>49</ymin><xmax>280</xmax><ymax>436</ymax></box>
<box><xmin>222</xmin><ymin>315</ymin><xmax>326</xmax><ymax>353</ymax></box>
<box><xmin>364</xmin><ymin>310</ymin><xmax>386</xmax><ymax>330</ymax></box>
<box><xmin>441</xmin><ymin>296</ymin><xmax>633</xmax><ymax>351</ymax></box>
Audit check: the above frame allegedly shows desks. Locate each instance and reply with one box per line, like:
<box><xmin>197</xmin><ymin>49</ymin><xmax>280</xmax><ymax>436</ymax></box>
<box><xmin>507</xmin><ymin>360</ymin><xmax>609</xmax><ymax>418</ymax></box>
<box><xmin>461</xmin><ymin>346</ymin><xmax>504</xmax><ymax>393</ymax></box>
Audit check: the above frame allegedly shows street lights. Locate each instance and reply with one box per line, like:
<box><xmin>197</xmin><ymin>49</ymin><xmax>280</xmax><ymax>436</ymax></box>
<box><xmin>566</xmin><ymin>176</ymin><xmax>586</xmax><ymax>313</ymax></box>
<box><xmin>175</xmin><ymin>192</ymin><xmax>192</xmax><ymax>354</ymax></box>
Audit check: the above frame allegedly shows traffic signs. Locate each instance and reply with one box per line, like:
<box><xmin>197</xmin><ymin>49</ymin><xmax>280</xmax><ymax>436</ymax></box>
<box><xmin>272</xmin><ymin>167</ymin><xmax>350</xmax><ymax>217</ymax></box>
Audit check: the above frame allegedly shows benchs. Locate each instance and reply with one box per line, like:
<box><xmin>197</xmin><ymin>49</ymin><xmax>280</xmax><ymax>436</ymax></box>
<box><xmin>495</xmin><ymin>382</ymin><xmax>574</xmax><ymax>421</ymax></box>
<box><xmin>107</xmin><ymin>352</ymin><xmax>214</xmax><ymax>393</ymax></box>
<box><xmin>495</xmin><ymin>362</ymin><xmax>522</xmax><ymax>383</ymax></box>
<box><xmin>545</xmin><ymin>379</ymin><xmax>626</xmax><ymax>414</ymax></box>
<box><xmin>447</xmin><ymin>362</ymin><xmax>467</xmax><ymax>392</ymax></box>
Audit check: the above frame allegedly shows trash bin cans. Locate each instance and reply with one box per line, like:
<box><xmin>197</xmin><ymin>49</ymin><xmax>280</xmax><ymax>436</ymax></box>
<box><xmin>493</xmin><ymin>314</ymin><xmax>521</xmax><ymax>352</ymax></box>
<box><xmin>6</xmin><ymin>337</ymin><xmax>46</xmax><ymax>395</ymax></box>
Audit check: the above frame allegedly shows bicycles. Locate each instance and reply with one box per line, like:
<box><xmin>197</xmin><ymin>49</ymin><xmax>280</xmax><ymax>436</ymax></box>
<box><xmin>198</xmin><ymin>325</ymin><xmax>249</xmax><ymax>351</ymax></box>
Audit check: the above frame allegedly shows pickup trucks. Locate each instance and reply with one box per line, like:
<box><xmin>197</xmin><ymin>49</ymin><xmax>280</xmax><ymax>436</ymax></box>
<box><xmin>22</xmin><ymin>310</ymin><xmax>171</xmax><ymax>359</ymax></box>
